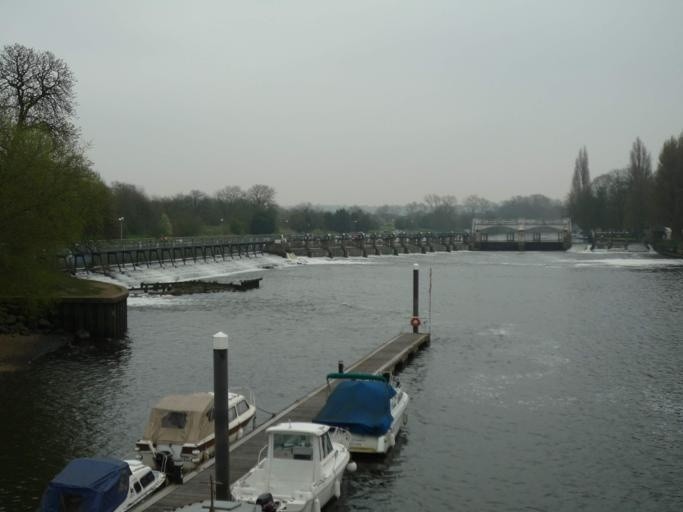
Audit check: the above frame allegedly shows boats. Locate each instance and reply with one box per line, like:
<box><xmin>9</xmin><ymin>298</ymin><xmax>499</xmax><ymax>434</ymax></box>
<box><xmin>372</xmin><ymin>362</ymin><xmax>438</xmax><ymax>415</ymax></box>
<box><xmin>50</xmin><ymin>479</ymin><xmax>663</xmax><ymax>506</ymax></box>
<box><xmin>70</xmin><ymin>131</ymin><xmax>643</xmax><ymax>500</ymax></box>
<box><xmin>36</xmin><ymin>451</ymin><xmax>165</xmax><ymax>512</ymax></box>
<box><xmin>314</xmin><ymin>369</ymin><xmax>412</xmax><ymax>459</ymax></box>
<box><xmin>133</xmin><ymin>388</ymin><xmax>259</xmax><ymax>468</ymax></box>
<box><xmin>149</xmin><ymin>477</ymin><xmax>289</xmax><ymax>512</ymax></box>
<box><xmin>227</xmin><ymin>421</ymin><xmax>354</xmax><ymax>510</ymax></box>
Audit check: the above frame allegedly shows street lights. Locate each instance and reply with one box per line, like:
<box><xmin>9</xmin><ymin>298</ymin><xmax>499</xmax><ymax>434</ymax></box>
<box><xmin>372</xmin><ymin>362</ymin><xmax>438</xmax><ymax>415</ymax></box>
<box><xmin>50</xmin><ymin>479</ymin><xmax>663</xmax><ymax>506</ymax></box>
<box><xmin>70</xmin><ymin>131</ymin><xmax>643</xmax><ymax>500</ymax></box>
<box><xmin>117</xmin><ymin>216</ymin><xmax>125</xmax><ymax>241</ymax></box>
<box><xmin>211</xmin><ymin>330</ymin><xmax>230</xmax><ymax>500</ymax></box>
<box><xmin>412</xmin><ymin>262</ymin><xmax>419</xmax><ymax>333</ymax></box>
